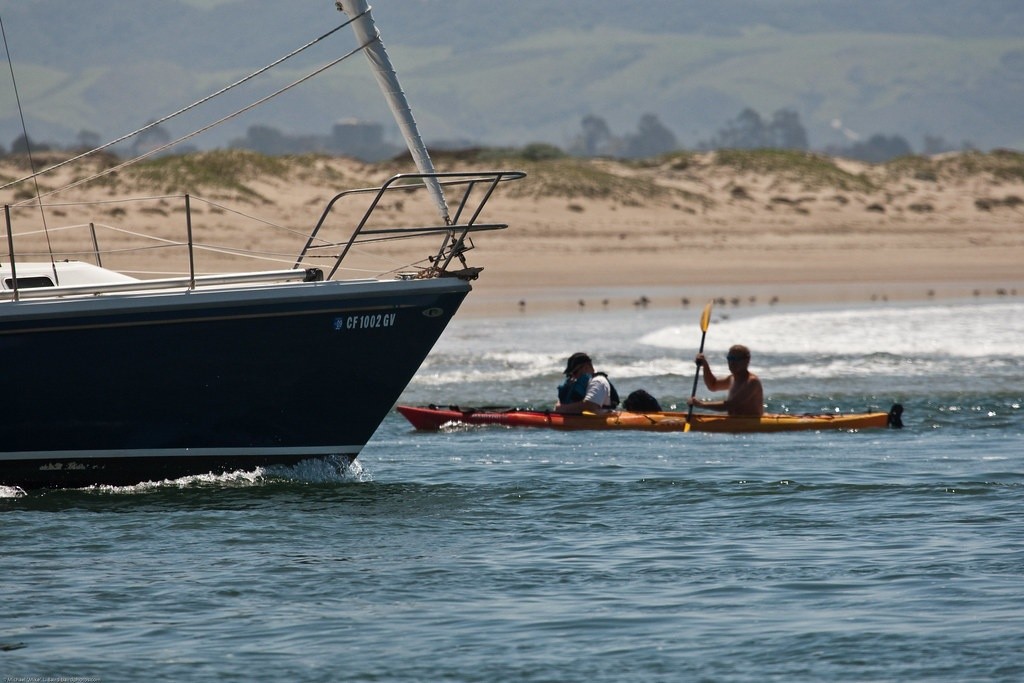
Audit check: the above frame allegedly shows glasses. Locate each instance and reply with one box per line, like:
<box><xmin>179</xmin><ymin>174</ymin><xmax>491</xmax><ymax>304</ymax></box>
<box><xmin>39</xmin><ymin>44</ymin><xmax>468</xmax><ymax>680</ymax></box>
<box><xmin>727</xmin><ymin>356</ymin><xmax>749</xmax><ymax>361</ymax></box>
<box><xmin>565</xmin><ymin>363</ymin><xmax>587</xmax><ymax>379</ymax></box>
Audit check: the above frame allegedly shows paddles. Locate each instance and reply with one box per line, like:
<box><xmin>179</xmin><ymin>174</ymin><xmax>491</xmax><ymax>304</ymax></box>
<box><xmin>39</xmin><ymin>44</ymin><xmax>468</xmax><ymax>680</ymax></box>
<box><xmin>683</xmin><ymin>299</ymin><xmax>714</xmax><ymax>432</ymax></box>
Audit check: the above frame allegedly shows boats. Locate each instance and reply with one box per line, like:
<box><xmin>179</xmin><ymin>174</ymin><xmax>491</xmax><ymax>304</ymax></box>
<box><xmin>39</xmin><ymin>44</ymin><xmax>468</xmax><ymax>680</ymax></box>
<box><xmin>395</xmin><ymin>390</ymin><xmax>906</xmax><ymax>436</ymax></box>
<box><xmin>0</xmin><ymin>1</ymin><xmax>525</xmax><ymax>498</ymax></box>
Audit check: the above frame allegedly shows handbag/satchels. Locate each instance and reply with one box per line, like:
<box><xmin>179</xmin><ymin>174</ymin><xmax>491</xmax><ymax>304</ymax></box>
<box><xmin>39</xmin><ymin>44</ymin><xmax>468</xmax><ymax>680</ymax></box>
<box><xmin>623</xmin><ymin>389</ymin><xmax>663</xmax><ymax>412</ymax></box>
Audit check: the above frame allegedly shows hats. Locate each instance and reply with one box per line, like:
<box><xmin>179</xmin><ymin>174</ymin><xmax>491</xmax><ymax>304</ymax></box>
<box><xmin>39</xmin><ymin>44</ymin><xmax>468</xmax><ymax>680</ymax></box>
<box><xmin>563</xmin><ymin>352</ymin><xmax>591</xmax><ymax>374</ymax></box>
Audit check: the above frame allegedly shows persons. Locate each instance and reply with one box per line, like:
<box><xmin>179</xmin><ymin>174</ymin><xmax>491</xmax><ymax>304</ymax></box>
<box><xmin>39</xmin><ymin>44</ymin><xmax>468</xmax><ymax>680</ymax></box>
<box><xmin>688</xmin><ymin>345</ymin><xmax>764</xmax><ymax>418</ymax></box>
<box><xmin>555</xmin><ymin>353</ymin><xmax>611</xmax><ymax>412</ymax></box>
